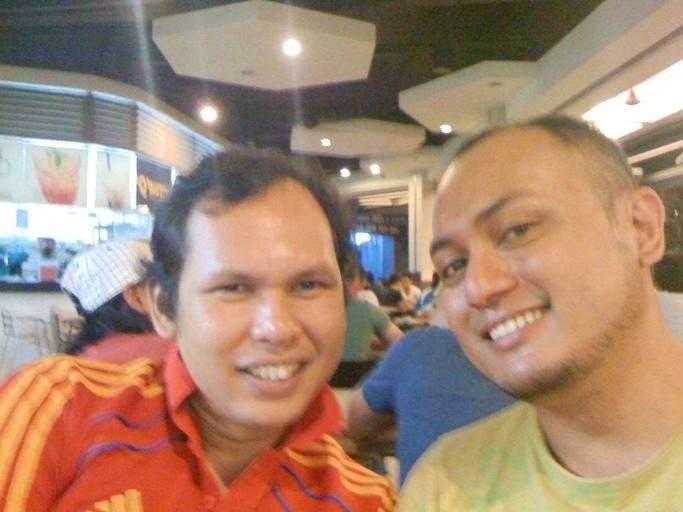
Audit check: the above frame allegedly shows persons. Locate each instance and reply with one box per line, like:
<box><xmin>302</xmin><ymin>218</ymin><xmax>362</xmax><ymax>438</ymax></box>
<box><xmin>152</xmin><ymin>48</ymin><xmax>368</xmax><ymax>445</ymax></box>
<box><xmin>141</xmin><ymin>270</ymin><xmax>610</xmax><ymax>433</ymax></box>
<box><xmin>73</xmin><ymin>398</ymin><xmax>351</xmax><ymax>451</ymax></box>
<box><xmin>396</xmin><ymin>111</ymin><xmax>683</xmax><ymax>512</ymax></box>
<box><xmin>345</xmin><ymin>325</ymin><xmax>521</xmax><ymax>490</ymax></box>
<box><xmin>0</xmin><ymin>143</ymin><xmax>396</xmax><ymax>512</ymax></box>
<box><xmin>56</xmin><ymin>236</ymin><xmax>161</xmax><ymax>358</ymax></box>
<box><xmin>329</xmin><ymin>245</ymin><xmax>440</xmax><ymax>388</ymax></box>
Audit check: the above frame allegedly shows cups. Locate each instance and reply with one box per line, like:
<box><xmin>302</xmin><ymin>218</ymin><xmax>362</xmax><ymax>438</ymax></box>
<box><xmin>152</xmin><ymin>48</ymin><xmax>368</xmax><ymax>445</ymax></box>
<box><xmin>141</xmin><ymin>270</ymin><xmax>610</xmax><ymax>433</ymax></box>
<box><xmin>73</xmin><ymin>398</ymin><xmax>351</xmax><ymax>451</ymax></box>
<box><xmin>29</xmin><ymin>147</ymin><xmax>128</xmax><ymax>208</ymax></box>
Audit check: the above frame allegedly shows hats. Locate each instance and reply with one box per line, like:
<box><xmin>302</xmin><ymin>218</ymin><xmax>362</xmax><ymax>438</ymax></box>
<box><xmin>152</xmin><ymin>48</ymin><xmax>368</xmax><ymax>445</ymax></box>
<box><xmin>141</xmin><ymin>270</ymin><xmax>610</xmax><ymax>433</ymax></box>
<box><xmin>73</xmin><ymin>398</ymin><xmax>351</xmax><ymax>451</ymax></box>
<box><xmin>58</xmin><ymin>237</ymin><xmax>155</xmax><ymax>316</ymax></box>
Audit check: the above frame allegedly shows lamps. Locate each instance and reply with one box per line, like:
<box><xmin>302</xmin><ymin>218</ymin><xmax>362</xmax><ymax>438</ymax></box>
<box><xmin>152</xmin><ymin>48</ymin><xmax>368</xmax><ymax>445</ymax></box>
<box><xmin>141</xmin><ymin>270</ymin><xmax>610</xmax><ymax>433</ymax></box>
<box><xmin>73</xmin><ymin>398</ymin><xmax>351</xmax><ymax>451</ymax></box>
<box><xmin>625</xmin><ymin>87</ymin><xmax>640</xmax><ymax>105</ymax></box>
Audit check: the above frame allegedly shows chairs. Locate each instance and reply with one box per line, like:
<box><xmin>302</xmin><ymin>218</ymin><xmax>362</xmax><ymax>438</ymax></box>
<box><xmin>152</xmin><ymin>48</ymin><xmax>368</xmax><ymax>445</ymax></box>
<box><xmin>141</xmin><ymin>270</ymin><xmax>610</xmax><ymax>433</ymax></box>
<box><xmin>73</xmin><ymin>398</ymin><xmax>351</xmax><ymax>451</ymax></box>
<box><xmin>1</xmin><ymin>303</ymin><xmax>82</xmax><ymax>375</ymax></box>
